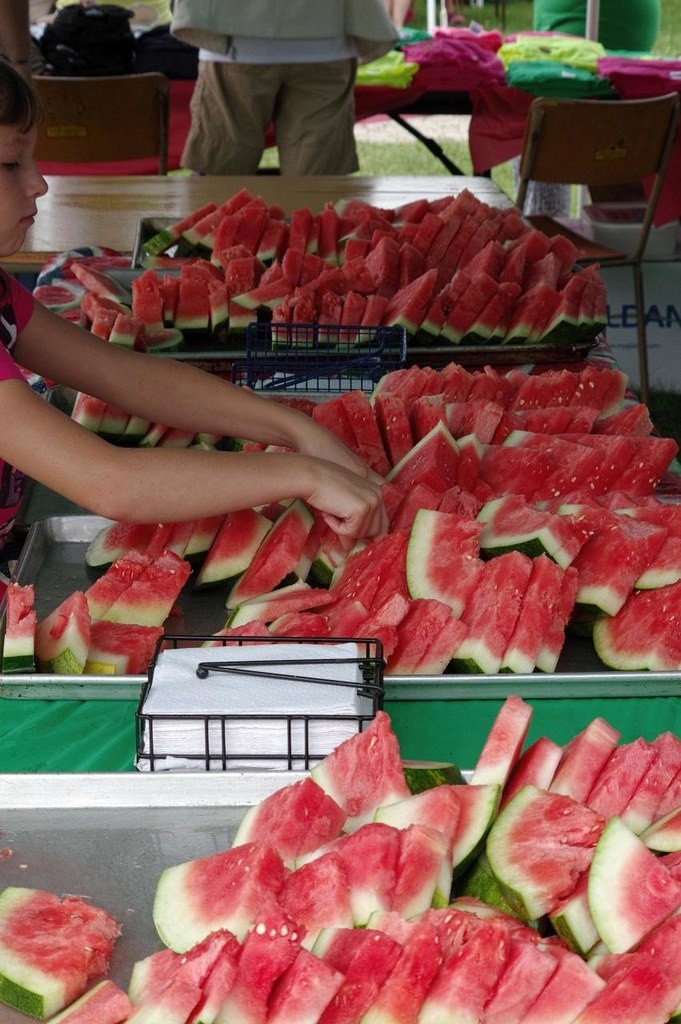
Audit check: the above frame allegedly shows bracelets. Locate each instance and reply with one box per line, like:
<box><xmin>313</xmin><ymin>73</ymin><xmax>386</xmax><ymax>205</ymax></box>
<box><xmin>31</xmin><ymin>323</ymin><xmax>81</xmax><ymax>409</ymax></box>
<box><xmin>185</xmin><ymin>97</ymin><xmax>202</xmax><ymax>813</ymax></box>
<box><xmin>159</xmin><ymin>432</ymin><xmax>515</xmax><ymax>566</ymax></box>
<box><xmin>0</xmin><ymin>53</ymin><xmax>29</xmax><ymax>64</ymax></box>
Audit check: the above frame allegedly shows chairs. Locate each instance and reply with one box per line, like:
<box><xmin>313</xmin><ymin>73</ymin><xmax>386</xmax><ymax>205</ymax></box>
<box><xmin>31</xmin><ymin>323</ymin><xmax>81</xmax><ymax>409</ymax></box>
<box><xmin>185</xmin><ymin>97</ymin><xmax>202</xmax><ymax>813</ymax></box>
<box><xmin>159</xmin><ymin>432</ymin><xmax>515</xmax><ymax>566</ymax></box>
<box><xmin>22</xmin><ymin>72</ymin><xmax>170</xmax><ymax>176</ymax></box>
<box><xmin>514</xmin><ymin>91</ymin><xmax>681</xmax><ymax>404</ymax></box>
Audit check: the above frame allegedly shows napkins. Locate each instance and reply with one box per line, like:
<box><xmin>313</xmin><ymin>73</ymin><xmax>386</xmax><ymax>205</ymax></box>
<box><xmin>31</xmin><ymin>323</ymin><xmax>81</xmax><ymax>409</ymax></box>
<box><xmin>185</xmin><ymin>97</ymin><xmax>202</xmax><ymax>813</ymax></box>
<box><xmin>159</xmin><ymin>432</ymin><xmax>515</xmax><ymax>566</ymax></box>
<box><xmin>133</xmin><ymin>642</ymin><xmax>369</xmax><ymax>772</ymax></box>
<box><xmin>254</xmin><ymin>371</ymin><xmax>377</xmax><ymax>391</ymax></box>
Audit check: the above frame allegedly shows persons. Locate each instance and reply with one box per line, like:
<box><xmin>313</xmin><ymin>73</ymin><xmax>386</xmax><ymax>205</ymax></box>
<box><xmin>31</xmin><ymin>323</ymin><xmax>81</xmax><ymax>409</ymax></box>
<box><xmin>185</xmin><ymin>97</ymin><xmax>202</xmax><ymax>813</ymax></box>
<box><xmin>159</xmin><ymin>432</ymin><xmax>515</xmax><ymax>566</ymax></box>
<box><xmin>0</xmin><ymin>0</ymin><xmax>30</xmax><ymax>85</ymax></box>
<box><xmin>383</xmin><ymin>0</ymin><xmax>486</xmax><ymax>36</ymax></box>
<box><xmin>180</xmin><ymin>0</ymin><xmax>359</xmax><ymax>176</ymax></box>
<box><xmin>1</xmin><ymin>56</ymin><xmax>392</xmax><ymax>559</ymax></box>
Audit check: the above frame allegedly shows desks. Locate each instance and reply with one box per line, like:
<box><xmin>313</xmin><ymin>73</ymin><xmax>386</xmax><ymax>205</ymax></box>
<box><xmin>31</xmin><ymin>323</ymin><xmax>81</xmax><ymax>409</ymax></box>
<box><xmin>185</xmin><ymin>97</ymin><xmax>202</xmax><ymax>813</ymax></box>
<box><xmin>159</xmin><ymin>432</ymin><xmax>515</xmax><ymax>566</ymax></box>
<box><xmin>169</xmin><ymin>89</ymin><xmax>678</xmax><ymax>177</ymax></box>
<box><xmin>2</xmin><ymin>354</ymin><xmax>681</xmax><ymax>774</ymax></box>
<box><xmin>0</xmin><ymin>175</ymin><xmax>535</xmax><ymax>273</ymax></box>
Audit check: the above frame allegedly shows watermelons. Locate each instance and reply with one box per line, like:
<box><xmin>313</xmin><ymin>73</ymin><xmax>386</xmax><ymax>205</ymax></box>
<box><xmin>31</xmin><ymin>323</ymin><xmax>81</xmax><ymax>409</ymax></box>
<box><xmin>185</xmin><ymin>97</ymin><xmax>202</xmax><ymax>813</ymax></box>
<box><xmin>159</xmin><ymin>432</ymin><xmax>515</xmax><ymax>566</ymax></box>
<box><xmin>0</xmin><ymin>191</ymin><xmax>681</xmax><ymax>1024</ymax></box>
